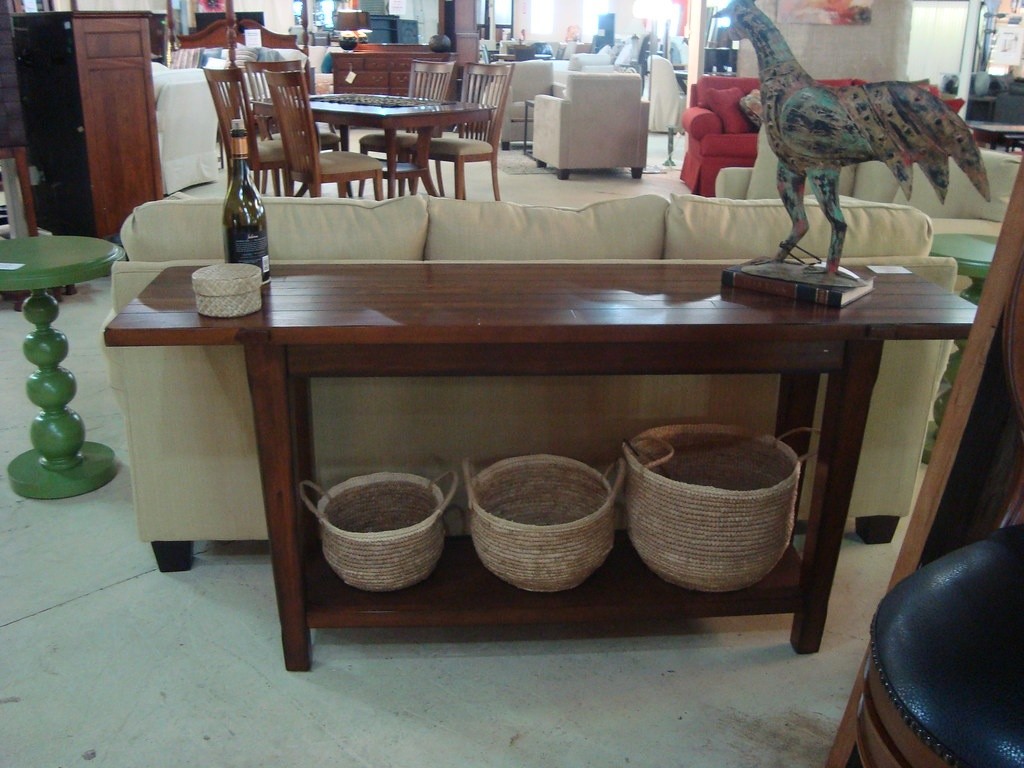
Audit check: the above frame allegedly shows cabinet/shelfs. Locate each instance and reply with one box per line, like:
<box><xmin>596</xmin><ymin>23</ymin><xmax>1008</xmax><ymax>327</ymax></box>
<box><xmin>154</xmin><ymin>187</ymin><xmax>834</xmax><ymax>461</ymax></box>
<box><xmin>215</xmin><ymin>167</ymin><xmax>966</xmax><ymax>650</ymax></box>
<box><xmin>330</xmin><ymin>44</ymin><xmax>463</xmax><ymax>129</ymax></box>
<box><xmin>11</xmin><ymin>12</ymin><xmax>165</xmax><ymax>239</ymax></box>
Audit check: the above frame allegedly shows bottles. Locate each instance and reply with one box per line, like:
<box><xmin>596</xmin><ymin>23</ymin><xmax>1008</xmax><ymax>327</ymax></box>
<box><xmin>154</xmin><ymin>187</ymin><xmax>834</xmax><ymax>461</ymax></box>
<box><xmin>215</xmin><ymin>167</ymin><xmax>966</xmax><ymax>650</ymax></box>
<box><xmin>221</xmin><ymin>128</ymin><xmax>270</xmax><ymax>289</ymax></box>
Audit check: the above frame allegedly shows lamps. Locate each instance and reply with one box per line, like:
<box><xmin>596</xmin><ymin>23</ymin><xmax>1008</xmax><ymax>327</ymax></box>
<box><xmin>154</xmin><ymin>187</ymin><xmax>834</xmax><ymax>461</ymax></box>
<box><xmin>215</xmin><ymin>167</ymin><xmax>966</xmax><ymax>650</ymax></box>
<box><xmin>333</xmin><ymin>11</ymin><xmax>374</xmax><ymax>50</ymax></box>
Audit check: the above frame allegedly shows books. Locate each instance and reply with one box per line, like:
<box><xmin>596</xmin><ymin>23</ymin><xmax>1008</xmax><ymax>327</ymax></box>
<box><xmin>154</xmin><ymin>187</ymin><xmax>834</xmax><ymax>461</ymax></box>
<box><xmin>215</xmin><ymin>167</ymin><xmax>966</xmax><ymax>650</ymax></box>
<box><xmin>721</xmin><ymin>256</ymin><xmax>877</xmax><ymax>308</ymax></box>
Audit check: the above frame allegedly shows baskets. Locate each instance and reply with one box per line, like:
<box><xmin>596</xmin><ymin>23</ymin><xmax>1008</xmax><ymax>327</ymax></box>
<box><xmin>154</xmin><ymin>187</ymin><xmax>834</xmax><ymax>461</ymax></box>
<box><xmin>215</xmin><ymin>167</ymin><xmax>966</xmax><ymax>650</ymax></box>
<box><xmin>621</xmin><ymin>422</ymin><xmax>826</xmax><ymax>593</ymax></box>
<box><xmin>298</xmin><ymin>458</ymin><xmax>457</xmax><ymax>593</ymax></box>
<box><xmin>461</xmin><ymin>456</ymin><xmax>626</xmax><ymax>593</ymax></box>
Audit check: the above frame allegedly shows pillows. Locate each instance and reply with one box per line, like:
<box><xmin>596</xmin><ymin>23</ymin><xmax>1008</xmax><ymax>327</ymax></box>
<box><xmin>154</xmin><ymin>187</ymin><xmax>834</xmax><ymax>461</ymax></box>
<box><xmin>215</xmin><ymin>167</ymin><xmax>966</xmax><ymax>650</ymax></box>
<box><xmin>556</xmin><ymin>42</ymin><xmax>577</xmax><ymax>60</ymax></box>
<box><xmin>171</xmin><ymin>48</ymin><xmax>308</xmax><ymax>68</ymax></box>
<box><xmin>705</xmin><ymin>87</ymin><xmax>764</xmax><ymax>134</ymax></box>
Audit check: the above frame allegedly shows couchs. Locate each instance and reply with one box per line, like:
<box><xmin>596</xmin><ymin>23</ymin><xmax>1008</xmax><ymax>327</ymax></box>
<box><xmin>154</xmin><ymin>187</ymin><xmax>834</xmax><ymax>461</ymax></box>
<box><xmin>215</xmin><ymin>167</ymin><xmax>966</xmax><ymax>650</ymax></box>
<box><xmin>966</xmin><ymin>71</ymin><xmax>1024</xmax><ymax>125</ymax></box>
<box><xmin>715</xmin><ymin>121</ymin><xmax>1022</xmax><ymax>237</ymax></box>
<box><xmin>680</xmin><ymin>74</ymin><xmax>964</xmax><ymax>191</ymax></box>
<box><xmin>482</xmin><ymin>33</ymin><xmax>649</xmax><ymax>179</ymax></box>
<box><xmin>103</xmin><ymin>201</ymin><xmax>934</xmax><ymax>573</ymax></box>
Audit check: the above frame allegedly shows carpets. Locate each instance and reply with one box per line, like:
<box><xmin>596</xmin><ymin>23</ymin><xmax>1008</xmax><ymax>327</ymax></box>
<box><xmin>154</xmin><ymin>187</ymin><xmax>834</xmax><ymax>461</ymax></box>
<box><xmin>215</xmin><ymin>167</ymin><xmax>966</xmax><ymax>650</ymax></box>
<box><xmin>497</xmin><ymin>143</ymin><xmax>667</xmax><ymax>174</ymax></box>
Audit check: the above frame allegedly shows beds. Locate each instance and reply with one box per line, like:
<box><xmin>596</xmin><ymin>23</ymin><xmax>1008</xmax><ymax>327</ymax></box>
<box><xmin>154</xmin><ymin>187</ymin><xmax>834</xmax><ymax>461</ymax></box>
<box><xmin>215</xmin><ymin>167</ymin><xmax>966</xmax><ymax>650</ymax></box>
<box><xmin>170</xmin><ymin>19</ymin><xmax>308</xmax><ymax>92</ymax></box>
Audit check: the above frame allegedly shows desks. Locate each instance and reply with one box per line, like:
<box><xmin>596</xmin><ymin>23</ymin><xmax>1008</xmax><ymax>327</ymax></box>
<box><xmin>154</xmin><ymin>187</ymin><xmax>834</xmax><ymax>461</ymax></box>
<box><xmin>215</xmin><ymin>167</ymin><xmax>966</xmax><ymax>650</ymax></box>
<box><xmin>250</xmin><ymin>93</ymin><xmax>498</xmax><ymax>200</ymax></box>
<box><xmin>968</xmin><ymin>126</ymin><xmax>1024</xmax><ymax>152</ymax></box>
<box><xmin>0</xmin><ymin>236</ymin><xmax>997</xmax><ymax>674</ymax></box>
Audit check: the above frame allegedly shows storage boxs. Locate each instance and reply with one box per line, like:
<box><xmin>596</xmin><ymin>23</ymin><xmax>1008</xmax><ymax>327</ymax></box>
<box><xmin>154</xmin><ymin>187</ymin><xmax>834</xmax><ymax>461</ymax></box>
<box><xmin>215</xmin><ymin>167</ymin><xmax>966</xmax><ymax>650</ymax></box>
<box><xmin>191</xmin><ymin>264</ymin><xmax>263</xmax><ymax>318</ymax></box>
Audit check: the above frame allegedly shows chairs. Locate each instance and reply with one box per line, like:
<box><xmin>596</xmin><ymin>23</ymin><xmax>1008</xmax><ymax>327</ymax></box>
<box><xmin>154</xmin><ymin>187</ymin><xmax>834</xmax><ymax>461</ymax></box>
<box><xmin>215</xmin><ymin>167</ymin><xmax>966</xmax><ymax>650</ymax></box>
<box><xmin>641</xmin><ymin>56</ymin><xmax>686</xmax><ymax>166</ymax></box>
<box><xmin>149</xmin><ymin>59</ymin><xmax>515</xmax><ymax>201</ymax></box>
<box><xmin>853</xmin><ymin>257</ymin><xmax>1024</xmax><ymax>768</ymax></box>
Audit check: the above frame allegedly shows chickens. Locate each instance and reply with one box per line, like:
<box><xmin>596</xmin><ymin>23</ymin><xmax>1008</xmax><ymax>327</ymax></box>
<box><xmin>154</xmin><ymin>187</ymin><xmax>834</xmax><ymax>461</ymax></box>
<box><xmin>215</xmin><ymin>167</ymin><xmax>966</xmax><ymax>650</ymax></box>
<box><xmin>710</xmin><ymin>0</ymin><xmax>991</xmax><ymax>288</ymax></box>
